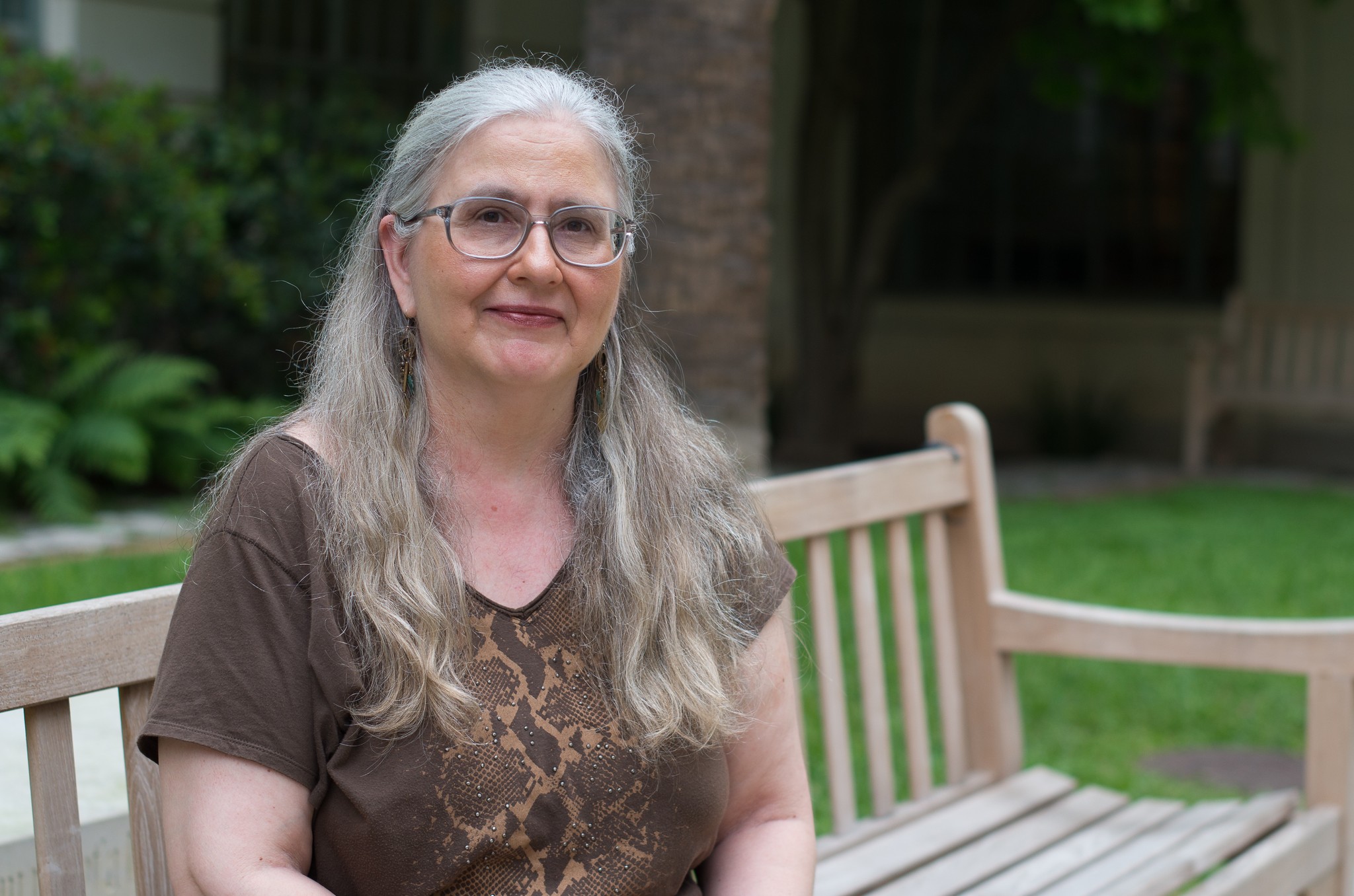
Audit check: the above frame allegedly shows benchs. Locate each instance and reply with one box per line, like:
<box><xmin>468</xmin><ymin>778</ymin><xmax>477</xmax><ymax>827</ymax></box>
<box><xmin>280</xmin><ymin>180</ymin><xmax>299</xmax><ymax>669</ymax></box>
<box><xmin>0</xmin><ymin>402</ymin><xmax>1353</xmax><ymax>896</ymax></box>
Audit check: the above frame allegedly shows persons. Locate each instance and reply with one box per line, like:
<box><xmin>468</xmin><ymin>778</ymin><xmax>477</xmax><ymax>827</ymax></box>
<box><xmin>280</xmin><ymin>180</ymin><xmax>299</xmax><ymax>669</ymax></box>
<box><xmin>138</xmin><ymin>64</ymin><xmax>820</xmax><ymax>896</ymax></box>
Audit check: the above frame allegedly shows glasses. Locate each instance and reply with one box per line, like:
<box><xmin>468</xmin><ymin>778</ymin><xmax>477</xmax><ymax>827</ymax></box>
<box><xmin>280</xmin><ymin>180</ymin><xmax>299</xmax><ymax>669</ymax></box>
<box><xmin>403</xmin><ymin>197</ymin><xmax>631</xmax><ymax>268</ymax></box>
